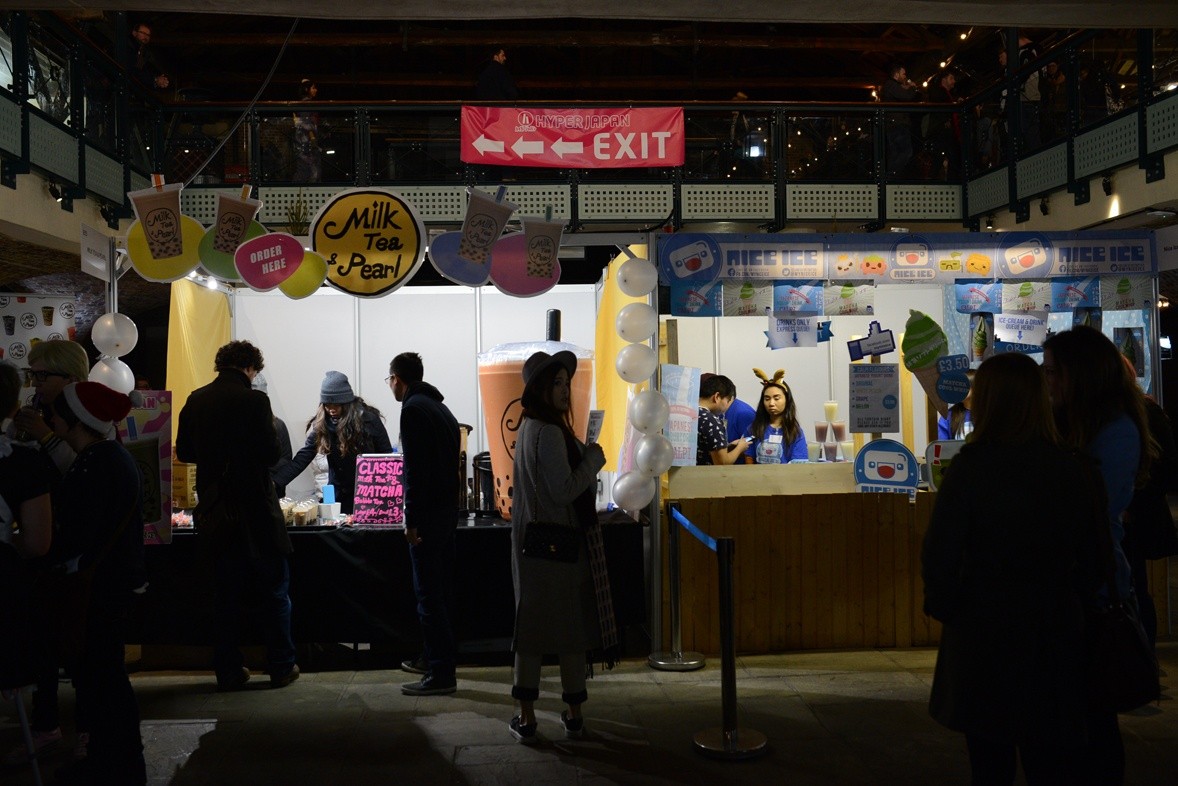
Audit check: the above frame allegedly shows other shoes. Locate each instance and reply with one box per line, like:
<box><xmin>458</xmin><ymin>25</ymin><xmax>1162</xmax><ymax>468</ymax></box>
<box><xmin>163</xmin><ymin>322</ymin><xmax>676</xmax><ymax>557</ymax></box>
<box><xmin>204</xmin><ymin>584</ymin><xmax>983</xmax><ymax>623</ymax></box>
<box><xmin>215</xmin><ymin>667</ymin><xmax>251</xmax><ymax>689</ymax></box>
<box><xmin>52</xmin><ymin>753</ymin><xmax>147</xmax><ymax>786</ymax></box>
<box><xmin>272</xmin><ymin>662</ymin><xmax>300</xmax><ymax>687</ymax></box>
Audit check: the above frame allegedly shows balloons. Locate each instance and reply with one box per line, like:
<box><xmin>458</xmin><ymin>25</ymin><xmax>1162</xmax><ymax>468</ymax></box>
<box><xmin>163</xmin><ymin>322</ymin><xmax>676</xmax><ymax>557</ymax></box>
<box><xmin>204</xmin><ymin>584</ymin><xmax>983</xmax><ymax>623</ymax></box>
<box><xmin>610</xmin><ymin>258</ymin><xmax>674</xmax><ymax>513</ymax></box>
<box><xmin>87</xmin><ymin>312</ymin><xmax>137</xmax><ymax>396</ymax></box>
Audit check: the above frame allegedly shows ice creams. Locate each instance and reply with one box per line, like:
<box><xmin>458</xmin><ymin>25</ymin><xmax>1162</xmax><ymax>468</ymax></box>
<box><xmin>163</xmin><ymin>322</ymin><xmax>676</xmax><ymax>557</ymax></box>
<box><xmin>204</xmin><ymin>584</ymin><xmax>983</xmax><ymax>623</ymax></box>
<box><xmin>971</xmin><ymin>317</ymin><xmax>988</xmax><ymax>359</ymax></box>
<box><xmin>900</xmin><ymin>308</ymin><xmax>948</xmax><ymax>420</ymax></box>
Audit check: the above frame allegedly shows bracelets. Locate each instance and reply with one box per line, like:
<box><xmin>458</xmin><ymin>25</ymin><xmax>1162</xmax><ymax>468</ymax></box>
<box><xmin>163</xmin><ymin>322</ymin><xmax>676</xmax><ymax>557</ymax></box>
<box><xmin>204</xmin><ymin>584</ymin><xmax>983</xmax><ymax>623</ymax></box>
<box><xmin>40</xmin><ymin>431</ymin><xmax>55</xmax><ymax>442</ymax></box>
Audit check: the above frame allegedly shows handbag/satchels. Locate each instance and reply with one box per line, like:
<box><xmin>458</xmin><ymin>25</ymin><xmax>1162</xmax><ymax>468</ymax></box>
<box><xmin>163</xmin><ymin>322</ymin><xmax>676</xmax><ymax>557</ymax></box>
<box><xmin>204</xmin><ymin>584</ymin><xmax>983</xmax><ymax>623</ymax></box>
<box><xmin>1140</xmin><ymin>490</ymin><xmax>1178</xmax><ymax>562</ymax></box>
<box><xmin>0</xmin><ymin>555</ymin><xmax>62</xmax><ymax>694</ymax></box>
<box><xmin>521</xmin><ymin>521</ymin><xmax>582</xmax><ymax>564</ymax></box>
<box><xmin>1098</xmin><ymin>601</ymin><xmax>1160</xmax><ymax>715</ymax></box>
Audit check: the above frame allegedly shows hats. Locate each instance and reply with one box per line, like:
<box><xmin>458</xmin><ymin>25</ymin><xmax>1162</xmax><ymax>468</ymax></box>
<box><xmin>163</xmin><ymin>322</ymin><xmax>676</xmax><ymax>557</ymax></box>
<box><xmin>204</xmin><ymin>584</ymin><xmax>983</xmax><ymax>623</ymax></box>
<box><xmin>521</xmin><ymin>349</ymin><xmax>577</xmax><ymax>409</ymax></box>
<box><xmin>319</xmin><ymin>370</ymin><xmax>354</xmax><ymax>404</ymax></box>
<box><xmin>249</xmin><ymin>371</ymin><xmax>267</xmax><ymax>395</ymax></box>
<box><xmin>64</xmin><ymin>381</ymin><xmax>144</xmax><ymax>435</ymax></box>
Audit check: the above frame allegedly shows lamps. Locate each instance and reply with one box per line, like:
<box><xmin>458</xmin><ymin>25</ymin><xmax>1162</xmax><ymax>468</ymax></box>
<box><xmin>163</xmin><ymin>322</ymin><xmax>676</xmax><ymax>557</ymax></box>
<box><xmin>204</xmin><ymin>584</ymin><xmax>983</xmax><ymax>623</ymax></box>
<box><xmin>49</xmin><ymin>185</ymin><xmax>63</xmax><ymax>202</ymax></box>
<box><xmin>99</xmin><ymin>206</ymin><xmax>111</xmax><ymax>221</ymax></box>
<box><xmin>1039</xmin><ymin>202</ymin><xmax>1048</xmax><ymax>216</ymax></box>
<box><xmin>985</xmin><ymin>220</ymin><xmax>993</xmax><ymax>229</ymax></box>
<box><xmin>1102</xmin><ymin>177</ymin><xmax>1111</xmax><ymax>197</ymax></box>
<box><xmin>741</xmin><ymin>129</ymin><xmax>768</xmax><ymax>163</ymax></box>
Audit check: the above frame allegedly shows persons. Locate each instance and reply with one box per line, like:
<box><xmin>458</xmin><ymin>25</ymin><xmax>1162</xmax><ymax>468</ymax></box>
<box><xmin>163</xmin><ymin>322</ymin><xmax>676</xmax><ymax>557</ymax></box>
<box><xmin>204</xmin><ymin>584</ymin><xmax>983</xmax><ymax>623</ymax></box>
<box><xmin>9</xmin><ymin>339</ymin><xmax>116</xmax><ymax>481</ymax></box>
<box><xmin>34</xmin><ymin>381</ymin><xmax>148</xmax><ymax>785</ymax></box>
<box><xmin>744</xmin><ymin>368</ymin><xmax>808</xmax><ymax>464</ymax></box>
<box><xmin>937</xmin><ymin>369</ymin><xmax>977</xmax><ymax>442</ymax></box>
<box><xmin>1</xmin><ymin>363</ymin><xmax>54</xmax><ymax>786</ymax></box>
<box><xmin>508</xmin><ymin>351</ymin><xmax>620</xmax><ymax>745</ymax></box>
<box><xmin>923</xmin><ymin>355</ymin><xmax>1122</xmax><ymax>786</ymax></box>
<box><xmin>272</xmin><ymin>370</ymin><xmax>394</xmax><ymax>514</ymax></box>
<box><xmin>697</xmin><ymin>374</ymin><xmax>752</xmax><ymax>465</ymax></box>
<box><xmin>387</xmin><ymin>352</ymin><xmax>461</xmax><ymax>697</ymax></box>
<box><xmin>122</xmin><ymin>21</ymin><xmax>1178</xmax><ymax>180</ymax></box>
<box><xmin>1040</xmin><ymin>326</ymin><xmax>1159</xmax><ymax>548</ymax></box>
<box><xmin>250</xmin><ymin>372</ymin><xmax>291</xmax><ymax>498</ymax></box>
<box><xmin>717</xmin><ymin>376</ymin><xmax>759</xmax><ymax>464</ymax></box>
<box><xmin>175</xmin><ymin>339</ymin><xmax>299</xmax><ymax>692</ymax></box>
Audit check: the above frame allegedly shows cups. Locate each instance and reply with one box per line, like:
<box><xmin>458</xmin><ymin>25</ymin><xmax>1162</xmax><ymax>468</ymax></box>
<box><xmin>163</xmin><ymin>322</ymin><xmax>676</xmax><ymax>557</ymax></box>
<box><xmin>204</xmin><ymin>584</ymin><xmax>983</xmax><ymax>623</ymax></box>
<box><xmin>121</xmin><ymin>431</ymin><xmax>162</xmax><ymax>524</ymax></box>
<box><xmin>479</xmin><ymin>348</ymin><xmax>594</xmax><ymax>521</ymax></box>
<box><xmin>807</xmin><ymin>400</ymin><xmax>854</xmax><ymax>463</ymax></box>
<box><xmin>279</xmin><ymin>494</ymin><xmax>318</xmax><ymax>527</ymax></box>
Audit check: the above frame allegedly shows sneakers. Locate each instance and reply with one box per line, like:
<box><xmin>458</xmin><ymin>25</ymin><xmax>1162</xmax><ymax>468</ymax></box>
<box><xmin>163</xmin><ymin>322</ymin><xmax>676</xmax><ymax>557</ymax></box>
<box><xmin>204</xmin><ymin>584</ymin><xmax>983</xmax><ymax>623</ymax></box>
<box><xmin>560</xmin><ymin>710</ymin><xmax>584</xmax><ymax>737</ymax></box>
<box><xmin>507</xmin><ymin>715</ymin><xmax>538</xmax><ymax>744</ymax></box>
<box><xmin>6</xmin><ymin>726</ymin><xmax>62</xmax><ymax>765</ymax></box>
<box><xmin>400</xmin><ymin>672</ymin><xmax>457</xmax><ymax>695</ymax></box>
<box><xmin>72</xmin><ymin>730</ymin><xmax>90</xmax><ymax>761</ymax></box>
<box><xmin>399</xmin><ymin>658</ymin><xmax>425</xmax><ymax>674</ymax></box>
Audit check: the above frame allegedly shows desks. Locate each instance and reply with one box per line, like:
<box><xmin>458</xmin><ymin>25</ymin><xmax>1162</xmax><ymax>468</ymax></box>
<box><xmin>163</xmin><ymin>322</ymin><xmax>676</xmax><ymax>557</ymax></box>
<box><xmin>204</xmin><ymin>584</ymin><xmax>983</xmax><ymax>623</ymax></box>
<box><xmin>128</xmin><ymin>505</ymin><xmax>654</xmax><ymax>660</ymax></box>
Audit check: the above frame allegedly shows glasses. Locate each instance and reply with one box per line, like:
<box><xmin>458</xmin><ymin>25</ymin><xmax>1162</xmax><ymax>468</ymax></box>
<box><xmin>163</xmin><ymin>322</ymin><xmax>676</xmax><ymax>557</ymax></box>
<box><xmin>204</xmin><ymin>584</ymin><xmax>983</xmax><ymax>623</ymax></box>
<box><xmin>384</xmin><ymin>375</ymin><xmax>395</xmax><ymax>385</ymax></box>
<box><xmin>26</xmin><ymin>368</ymin><xmax>70</xmax><ymax>383</ymax></box>
